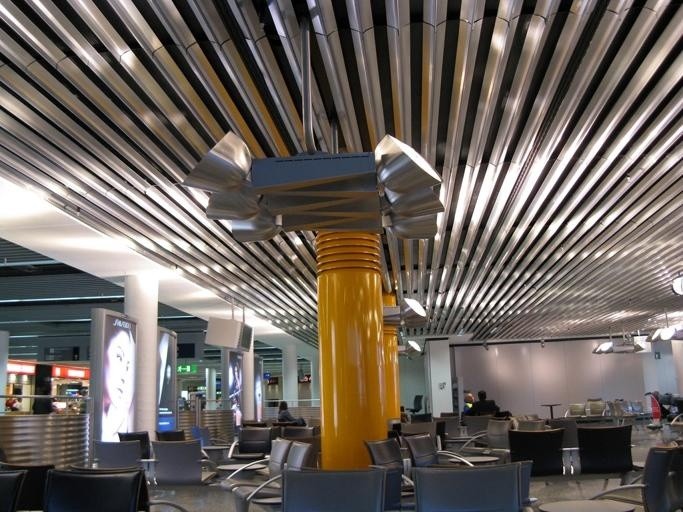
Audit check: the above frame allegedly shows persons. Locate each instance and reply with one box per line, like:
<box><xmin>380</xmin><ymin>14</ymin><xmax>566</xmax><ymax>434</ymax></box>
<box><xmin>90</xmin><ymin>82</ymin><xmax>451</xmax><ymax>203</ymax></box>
<box><xmin>475</xmin><ymin>391</ymin><xmax>502</xmax><ymax>411</ymax></box>
<box><xmin>230</xmin><ymin>362</ymin><xmax>242</xmax><ymax>427</ymax></box>
<box><xmin>652</xmin><ymin>390</ymin><xmax>679</xmax><ymax>422</ymax></box>
<box><xmin>101</xmin><ymin>325</ymin><xmax>134</xmax><ymax>439</ymax></box>
<box><xmin>463</xmin><ymin>393</ymin><xmax>478</xmax><ymax>415</ymax></box>
<box><xmin>5</xmin><ymin>388</ymin><xmax>90</xmax><ymax>412</ymax></box>
<box><xmin>278</xmin><ymin>400</ymin><xmax>295</xmax><ymax>423</ymax></box>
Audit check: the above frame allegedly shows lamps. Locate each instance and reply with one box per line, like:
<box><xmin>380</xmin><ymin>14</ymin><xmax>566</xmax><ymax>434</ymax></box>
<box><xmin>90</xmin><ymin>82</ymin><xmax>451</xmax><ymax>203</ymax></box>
<box><xmin>383</xmin><ymin>297</ymin><xmax>429</xmax><ymax>360</ymax></box>
<box><xmin>180</xmin><ymin>20</ymin><xmax>446</xmax><ymax>245</ymax></box>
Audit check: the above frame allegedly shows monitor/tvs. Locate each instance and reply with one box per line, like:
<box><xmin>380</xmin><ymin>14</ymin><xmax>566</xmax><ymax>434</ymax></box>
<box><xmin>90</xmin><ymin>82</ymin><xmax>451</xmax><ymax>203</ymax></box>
<box><xmin>204</xmin><ymin>316</ymin><xmax>252</xmax><ymax>352</ymax></box>
<box><xmin>269</xmin><ymin>377</ymin><xmax>278</xmax><ymax>385</ymax></box>
<box><xmin>298</xmin><ymin>375</ymin><xmax>311</xmax><ymax>383</ymax></box>
